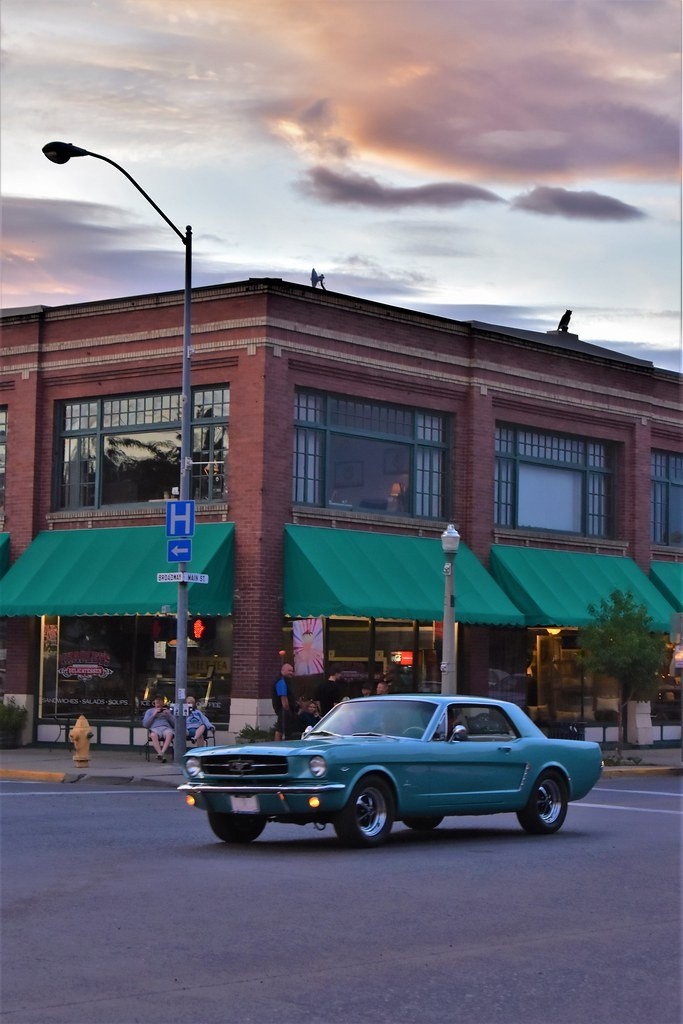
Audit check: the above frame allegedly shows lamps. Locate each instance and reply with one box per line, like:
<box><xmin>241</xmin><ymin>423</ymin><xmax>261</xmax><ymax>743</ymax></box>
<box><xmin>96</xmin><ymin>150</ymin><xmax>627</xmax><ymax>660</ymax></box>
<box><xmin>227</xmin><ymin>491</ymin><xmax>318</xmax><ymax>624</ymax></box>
<box><xmin>390</xmin><ymin>484</ymin><xmax>402</xmax><ymax>513</ymax></box>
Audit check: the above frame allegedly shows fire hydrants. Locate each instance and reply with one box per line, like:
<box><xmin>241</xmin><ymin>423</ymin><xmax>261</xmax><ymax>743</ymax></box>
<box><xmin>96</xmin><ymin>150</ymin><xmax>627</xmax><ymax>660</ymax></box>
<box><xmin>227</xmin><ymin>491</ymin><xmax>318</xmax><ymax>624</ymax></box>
<box><xmin>69</xmin><ymin>715</ymin><xmax>94</xmax><ymax>766</ymax></box>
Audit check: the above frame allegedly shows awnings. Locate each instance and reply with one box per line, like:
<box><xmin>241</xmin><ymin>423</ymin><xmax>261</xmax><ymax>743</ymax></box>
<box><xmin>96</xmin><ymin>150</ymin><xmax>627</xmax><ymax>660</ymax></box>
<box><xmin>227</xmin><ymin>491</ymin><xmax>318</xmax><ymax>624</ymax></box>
<box><xmin>282</xmin><ymin>523</ymin><xmax>683</xmax><ymax>627</ymax></box>
<box><xmin>0</xmin><ymin>521</ymin><xmax>236</xmax><ymax>617</ymax></box>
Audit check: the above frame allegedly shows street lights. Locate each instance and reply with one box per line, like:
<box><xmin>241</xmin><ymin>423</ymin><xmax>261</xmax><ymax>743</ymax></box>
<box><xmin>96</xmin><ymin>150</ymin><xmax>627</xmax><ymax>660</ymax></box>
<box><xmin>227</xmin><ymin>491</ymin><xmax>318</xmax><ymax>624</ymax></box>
<box><xmin>43</xmin><ymin>141</ymin><xmax>194</xmax><ymax>762</ymax></box>
<box><xmin>439</xmin><ymin>524</ymin><xmax>461</xmax><ymax>695</ymax></box>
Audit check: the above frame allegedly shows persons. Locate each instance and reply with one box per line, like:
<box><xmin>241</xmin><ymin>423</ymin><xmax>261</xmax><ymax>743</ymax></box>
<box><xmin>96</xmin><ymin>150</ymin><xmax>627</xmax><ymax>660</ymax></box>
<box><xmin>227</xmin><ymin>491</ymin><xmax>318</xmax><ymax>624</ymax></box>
<box><xmin>143</xmin><ymin>695</ymin><xmax>176</xmax><ymax>764</ymax></box>
<box><xmin>271</xmin><ymin>663</ymin><xmax>390</xmax><ymax>741</ymax></box>
<box><xmin>185</xmin><ymin>696</ymin><xmax>216</xmax><ymax>747</ymax></box>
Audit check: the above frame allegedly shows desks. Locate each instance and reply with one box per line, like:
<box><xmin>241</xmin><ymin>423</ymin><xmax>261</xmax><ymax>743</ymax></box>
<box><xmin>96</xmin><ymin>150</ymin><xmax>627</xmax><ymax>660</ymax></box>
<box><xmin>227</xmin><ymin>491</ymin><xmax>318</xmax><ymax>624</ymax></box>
<box><xmin>328</xmin><ymin>503</ymin><xmax>352</xmax><ymax>511</ymax></box>
<box><xmin>42</xmin><ymin>712</ymin><xmax>96</xmax><ymax>752</ymax></box>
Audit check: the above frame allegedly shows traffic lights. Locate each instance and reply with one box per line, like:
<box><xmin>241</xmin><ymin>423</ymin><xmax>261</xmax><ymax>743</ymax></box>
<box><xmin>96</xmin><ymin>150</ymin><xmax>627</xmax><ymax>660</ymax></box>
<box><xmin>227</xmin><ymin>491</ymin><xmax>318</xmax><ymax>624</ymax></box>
<box><xmin>188</xmin><ymin>611</ymin><xmax>217</xmax><ymax>649</ymax></box>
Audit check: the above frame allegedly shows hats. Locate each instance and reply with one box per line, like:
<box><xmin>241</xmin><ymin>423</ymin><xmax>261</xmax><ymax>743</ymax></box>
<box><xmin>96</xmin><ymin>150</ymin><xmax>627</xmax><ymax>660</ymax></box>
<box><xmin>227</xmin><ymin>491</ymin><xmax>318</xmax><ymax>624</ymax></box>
<box><xmin>154</xmin><ymin>694</ymin><xmax>164</xmax><ymax>700</ymax></box>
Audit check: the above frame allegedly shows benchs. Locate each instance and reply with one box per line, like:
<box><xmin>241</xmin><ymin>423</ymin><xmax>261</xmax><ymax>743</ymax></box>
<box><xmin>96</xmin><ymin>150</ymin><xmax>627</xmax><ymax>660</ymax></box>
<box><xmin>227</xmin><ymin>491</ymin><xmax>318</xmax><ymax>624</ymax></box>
<box><xmin>145</xmin><ymin>728</ymin><xmax>215</xmax><ymax>762</ymax></box>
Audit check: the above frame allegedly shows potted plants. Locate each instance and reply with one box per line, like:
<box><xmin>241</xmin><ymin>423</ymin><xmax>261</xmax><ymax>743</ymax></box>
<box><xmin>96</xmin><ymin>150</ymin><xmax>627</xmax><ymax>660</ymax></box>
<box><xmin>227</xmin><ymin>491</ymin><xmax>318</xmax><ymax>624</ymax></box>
<box><xmin>234</xmin><ymin>723</ymin><xmax>270</xmax><ymax>743</ymax></box>
<box><xmin>0</xmin><ymin>696</ymin><xmax>27</xmax><ymax>750</ymax></box>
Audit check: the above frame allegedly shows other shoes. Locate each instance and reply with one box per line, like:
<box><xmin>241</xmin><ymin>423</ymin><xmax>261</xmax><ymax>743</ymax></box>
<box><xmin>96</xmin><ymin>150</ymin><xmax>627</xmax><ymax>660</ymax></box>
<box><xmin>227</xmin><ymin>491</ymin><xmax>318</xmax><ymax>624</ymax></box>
<box><xmin>162</xmin><ymin>759</ymin><xmax>167</xmax><ymax>764</ymax></box>
<box><xmin>156</xmin><ymin>753</ymin><xmax>163</xmax><ymax>760</ymax></box>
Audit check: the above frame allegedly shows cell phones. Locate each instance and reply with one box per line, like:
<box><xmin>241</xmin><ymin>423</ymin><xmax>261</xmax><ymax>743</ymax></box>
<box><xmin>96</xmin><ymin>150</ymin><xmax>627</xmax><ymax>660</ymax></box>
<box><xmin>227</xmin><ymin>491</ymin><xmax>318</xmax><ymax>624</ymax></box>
<box><xmin>158</xmin><ymin>708</ymin><xmax>166</xmax><ymax>713</ymax></box>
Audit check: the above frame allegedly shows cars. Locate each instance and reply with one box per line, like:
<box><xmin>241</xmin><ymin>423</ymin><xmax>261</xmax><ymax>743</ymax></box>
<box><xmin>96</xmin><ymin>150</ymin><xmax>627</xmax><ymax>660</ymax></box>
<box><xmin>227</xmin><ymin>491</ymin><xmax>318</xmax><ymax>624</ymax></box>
<box><xmin>485</xmin><ymin>673</ymin><xmax>531</xmax><ymax>706</ymax></box>
<box><xmin>177</xmin><ymin>694</ymin><xmax>605</xmax><ymax>849</ymax></box>
